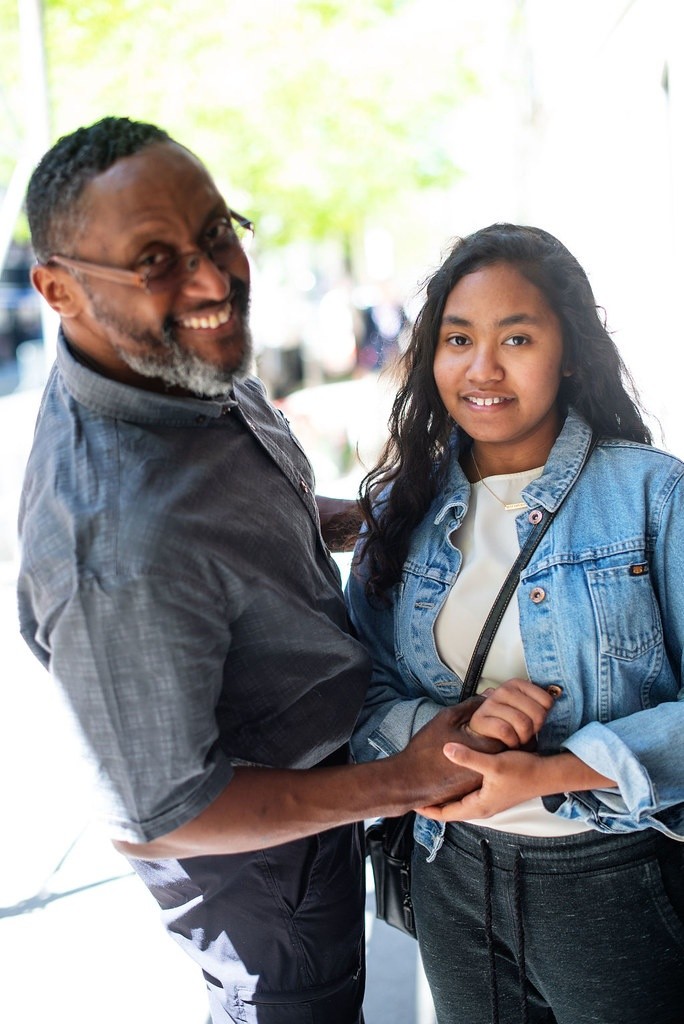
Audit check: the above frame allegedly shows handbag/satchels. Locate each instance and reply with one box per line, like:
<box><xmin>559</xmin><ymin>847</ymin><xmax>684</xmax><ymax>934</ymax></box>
<box><xmin>363</xmin><ymin>809</ymin><xmax>420</xmax><ymax>941</ymax></box>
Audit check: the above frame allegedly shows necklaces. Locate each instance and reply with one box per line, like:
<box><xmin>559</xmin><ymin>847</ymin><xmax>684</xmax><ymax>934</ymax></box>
<box><xmin>467</xmin><ymin>447</ymin><xmax>537</xmax><ymax>512</ymax></box>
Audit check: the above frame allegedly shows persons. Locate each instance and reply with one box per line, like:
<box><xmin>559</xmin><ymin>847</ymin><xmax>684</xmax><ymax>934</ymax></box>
<box><xmin>17</xmin><ymin>114</ymin><xmax>508</xmax><ymax>1024</ymax></box>
<box><xmin>349</xmin><ymin>223</ymin><xmax>684</xmax><ymax>1023</ymax></box>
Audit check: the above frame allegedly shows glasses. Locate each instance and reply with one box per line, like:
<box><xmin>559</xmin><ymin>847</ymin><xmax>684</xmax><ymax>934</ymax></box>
<box><xmin>43</xmin><ymin>209</ymin><xmax>255</xmax><ymax>296</ymax></box>
